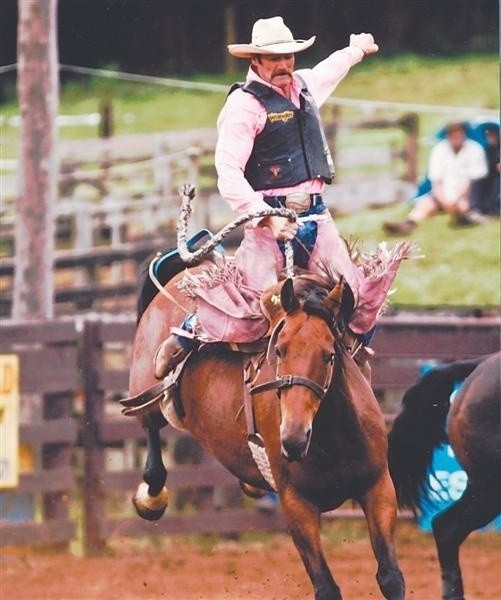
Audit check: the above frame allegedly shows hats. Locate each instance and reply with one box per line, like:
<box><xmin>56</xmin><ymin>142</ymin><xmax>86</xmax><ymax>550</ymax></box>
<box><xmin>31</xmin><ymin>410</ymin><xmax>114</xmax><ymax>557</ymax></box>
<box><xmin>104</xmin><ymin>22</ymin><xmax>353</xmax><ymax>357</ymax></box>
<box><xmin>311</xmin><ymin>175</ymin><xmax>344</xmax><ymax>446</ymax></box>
<box><xmin>228</xmin><ymin>16</ymin><xmax>319</xmax><ymax>57</ymax></box>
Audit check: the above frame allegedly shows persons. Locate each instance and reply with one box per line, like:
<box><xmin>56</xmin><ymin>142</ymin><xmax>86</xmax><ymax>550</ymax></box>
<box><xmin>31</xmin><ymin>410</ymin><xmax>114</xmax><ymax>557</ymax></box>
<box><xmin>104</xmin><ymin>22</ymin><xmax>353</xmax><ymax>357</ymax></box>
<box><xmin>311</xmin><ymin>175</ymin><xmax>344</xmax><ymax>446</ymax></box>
<box><xmin>469</xmin><ymin>123</ymin><xmax>501</xmax><ymax>217</ymax></box>
<box><xmin>382</xmin><ymin>121</ymin><xmax>488</xmax><ymax>234</ymax></box>
<box><xmin>150</xmin><ymin>14</ymin><xmax>381</xmax><ymax>385</ymax></box>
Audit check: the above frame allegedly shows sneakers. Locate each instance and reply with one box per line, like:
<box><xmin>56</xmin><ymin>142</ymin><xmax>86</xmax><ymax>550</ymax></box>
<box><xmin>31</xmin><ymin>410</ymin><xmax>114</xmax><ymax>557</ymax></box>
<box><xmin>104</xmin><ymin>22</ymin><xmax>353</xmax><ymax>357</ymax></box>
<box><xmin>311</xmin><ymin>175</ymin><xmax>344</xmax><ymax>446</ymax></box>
<box><xmin>455</xmin><ymin>210</ymin><xmax>476</xmax><ymax>226</ymax></box>
<box><xmin>384</xmin><ymin>220</ymin><xmax>412</xmax><ymax>236</ymax></box>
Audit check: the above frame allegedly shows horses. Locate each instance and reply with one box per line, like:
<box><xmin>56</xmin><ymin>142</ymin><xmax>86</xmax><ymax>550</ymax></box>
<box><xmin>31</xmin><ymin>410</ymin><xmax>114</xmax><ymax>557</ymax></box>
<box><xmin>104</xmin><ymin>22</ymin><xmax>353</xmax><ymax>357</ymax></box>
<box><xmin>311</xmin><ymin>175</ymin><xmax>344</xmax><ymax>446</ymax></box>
<box><xmin>130</xmin><ymin>247</ymin><xmax>405</xmax><ymax>600</ymax></box>
<box><xmin>387</xmin><ymin>352</ymin><xmax>501</xmax><ymax>600</ymax></box>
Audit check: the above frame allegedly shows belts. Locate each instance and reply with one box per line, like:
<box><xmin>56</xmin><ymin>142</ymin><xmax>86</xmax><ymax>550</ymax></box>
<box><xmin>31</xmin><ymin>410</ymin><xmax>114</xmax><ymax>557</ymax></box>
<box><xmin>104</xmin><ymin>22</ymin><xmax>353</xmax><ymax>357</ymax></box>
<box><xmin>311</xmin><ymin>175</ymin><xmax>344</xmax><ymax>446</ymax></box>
<box><xmin>264</xmin><ymin>193</ymin><xmax>323</xmax><ymax>209</ymax></box>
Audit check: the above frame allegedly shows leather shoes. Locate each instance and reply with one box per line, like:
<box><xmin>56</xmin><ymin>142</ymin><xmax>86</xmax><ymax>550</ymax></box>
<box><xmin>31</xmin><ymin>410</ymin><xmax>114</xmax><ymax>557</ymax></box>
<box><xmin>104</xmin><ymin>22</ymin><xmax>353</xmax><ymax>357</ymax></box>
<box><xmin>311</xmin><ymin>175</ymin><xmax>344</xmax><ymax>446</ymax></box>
<box><xmin>154</xmin><ymin>314</ymin><xmax>195</xmax><ymax>379</ymax></box>
